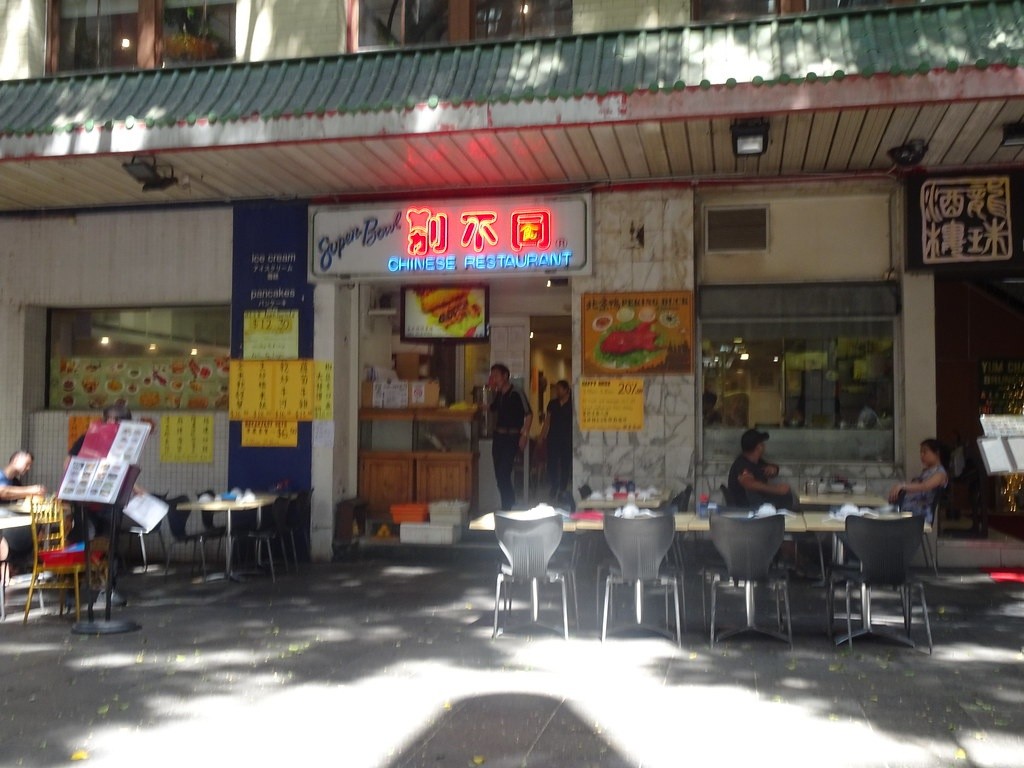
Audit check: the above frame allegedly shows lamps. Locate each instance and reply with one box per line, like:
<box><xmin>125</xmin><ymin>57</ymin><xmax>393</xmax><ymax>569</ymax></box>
<box><xmin>728</xmin><ymin>116</ymin><xmax>770</xmax><ymax>156</ymax></box>
<box><xmin>122</xmin><ymin>151</ymin><xmax>181</xmax><ymax>192</ymax></box>
<box><xmin>1001</xmin><ymin>123</ymin><xmax>1024</xmax><ymax>145</ymax></box>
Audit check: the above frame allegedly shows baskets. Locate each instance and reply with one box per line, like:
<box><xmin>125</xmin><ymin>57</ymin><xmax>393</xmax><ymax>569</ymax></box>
<box><xmin>389</xmin><ymin>503</ymin><xmax>469</xmax><ymax>545</ymax></box>
<box><xmin>39</xmin><ymin>548</ymin><xmax>103</xmax><ymax>568</ymax></box>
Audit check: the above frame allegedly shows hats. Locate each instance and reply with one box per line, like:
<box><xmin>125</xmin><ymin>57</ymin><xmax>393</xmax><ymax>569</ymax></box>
<box><xmin>741</xmin><ymin>429</ymin><xmax>769</xmax><ymax>450</ymax></box>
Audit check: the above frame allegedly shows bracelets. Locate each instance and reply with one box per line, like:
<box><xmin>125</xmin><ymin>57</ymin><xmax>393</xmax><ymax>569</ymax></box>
<box><xmin>523</xmin><ymin>430</ymin><xmax>528</xmax><ymax>436</ymax></box>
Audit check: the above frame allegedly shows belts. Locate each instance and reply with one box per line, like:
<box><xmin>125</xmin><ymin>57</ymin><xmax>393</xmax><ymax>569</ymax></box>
<box><xmin>496</xmin><ymin>428</ymin><xmax>520</xmax><ymax>434</ymax></box>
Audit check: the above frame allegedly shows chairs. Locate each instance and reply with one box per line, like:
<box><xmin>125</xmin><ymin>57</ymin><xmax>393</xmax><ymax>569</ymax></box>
<box><xmin>116</xmin><ymin>490</ymin><xmax>299</xmax><ymax>588</ymax></box>
<box><xmin>493</xmin><ymin>477</ymin><xmax>983</xmax><ymax>655</ymax></box>
<box><xmin>23</xmin><ymin>493</ymin><xmax>108</xmax><ymax>627</ymax></box>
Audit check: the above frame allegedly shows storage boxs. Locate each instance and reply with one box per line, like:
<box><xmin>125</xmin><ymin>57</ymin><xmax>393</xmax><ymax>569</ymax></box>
<box><xmin>360</xmin><ymin>349</ymin><xmax>440</xmax><ymax>408</ymax></box>
<box><xmin>389</xmin><ymin>502</ymin><xmax>470</xmax><ymax>544</ymax></box>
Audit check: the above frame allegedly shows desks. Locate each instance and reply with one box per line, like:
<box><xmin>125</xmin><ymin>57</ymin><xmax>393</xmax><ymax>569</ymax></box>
<box><xmin>469</xmin><ymin>512</ymin><xmax>575</xmax><ymax>615</ymax></box>
<box><xmin>804</xmin><ymin>511</ymin><xmax>913</xmax><ymax>621</ymax></box>
<box><xmin>800</xmin><ymin>493</ymin><xmax>889</xmax><ymax>535</ymax></box>
<box><xmin>177</xmin><ymin>497</ymin><xmax>276</xmax><ymax>577</ymax></box>
<box><xmin>688</xmin><ymin>509</ymin><xmax>805</xmax><ymax>636</ymax></box>
<box><xmin>578</xmin><ymin>489</ymin><xmax>671</xmax><ymax>509</ymax></box>
<box><xmin>0</xmin><ymin>502</ymin><xmax>70</xmax><ymax>619</ymax></box>
<box><xmin>578</xmin><ymin>512</ymin><xmax>686</xmax><ymax>625</ymax></box>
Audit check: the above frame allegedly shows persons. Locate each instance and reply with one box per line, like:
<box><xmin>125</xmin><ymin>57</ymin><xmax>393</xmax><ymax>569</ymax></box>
<box><xmin>888</xmin><ymin>438</ymin><xmax>949</xmax><ymax>525</ymax></box>
<box><xmin>489</xmin><ymin>364</ymin><xmax>534</xmax><ymax>511</ymax></box>
<box><xmin>0</xmin><ymin>449</ymin><xmax>45</xmax><ymax>575</ymax></box>
<box><xmin>704</xmin><ymin>392</ymin><xmax>723</xmax><ymax>426</ymax></box>
<box><xmin>62</xmin><ymin>405</ymin><xmax>145</xmax><ymax>606</ymax></box>
<box><xmin>966</xmin><ymin>441</ymin><xmax>995</xmax><ymax>537</ymax></box>
<box><xmin>726</xmin><ymin>429</ymin><xmax>809</xmax><ymax>570</ymax></box>
<box><xmin>538</xmin><ymin>382</ymin><xmax>571</xmax><ymax>500</ymax></box>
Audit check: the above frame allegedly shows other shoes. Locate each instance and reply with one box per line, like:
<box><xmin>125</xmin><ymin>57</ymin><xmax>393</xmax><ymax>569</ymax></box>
<box><xmin>558</xmin><ymin>489</ymin><xmax>565</xmax><ymax>499</ymax></box>
<box><xmin>549</xmin><ymin>486</ymin><xmax>557</xmax><ymax>496</ymax></box>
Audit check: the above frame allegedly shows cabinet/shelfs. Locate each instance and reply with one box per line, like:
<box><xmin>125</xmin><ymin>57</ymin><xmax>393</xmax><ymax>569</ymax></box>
<box><xmin>360</xmin><ymin>406</ymin><xmax>481</xmax><ymax>519</ymax></box>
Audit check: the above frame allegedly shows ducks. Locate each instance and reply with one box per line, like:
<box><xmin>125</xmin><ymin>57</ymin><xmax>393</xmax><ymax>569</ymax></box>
<box><xmin>598</xmin><ymin>319</ymin><xmax>666</xmax><ymax>355</ymax></box>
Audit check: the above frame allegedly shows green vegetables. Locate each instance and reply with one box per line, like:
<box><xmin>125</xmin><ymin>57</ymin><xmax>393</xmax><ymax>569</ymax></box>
<box><xmin>592</xmin><ymin>320</ymin><xmax>669</xmax><ymax>369</ymax></box>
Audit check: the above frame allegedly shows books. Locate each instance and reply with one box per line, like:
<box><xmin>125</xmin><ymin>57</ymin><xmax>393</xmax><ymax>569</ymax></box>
<box><xmin>57</xmin><ymin>418</ymin><xmax>152</xmax><ymax>505</ymax></box>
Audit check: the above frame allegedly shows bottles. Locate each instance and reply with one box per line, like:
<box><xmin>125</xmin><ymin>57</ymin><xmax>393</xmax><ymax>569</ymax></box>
<box><xmin>700</xmin><ymin>495</ymin><xmax>709</xmax><ymax>521</ymax></box>
<box><xmin>627</xmin><ymin>480</ymin><xmax>635</xmax><ymax>502</ymax></box>
